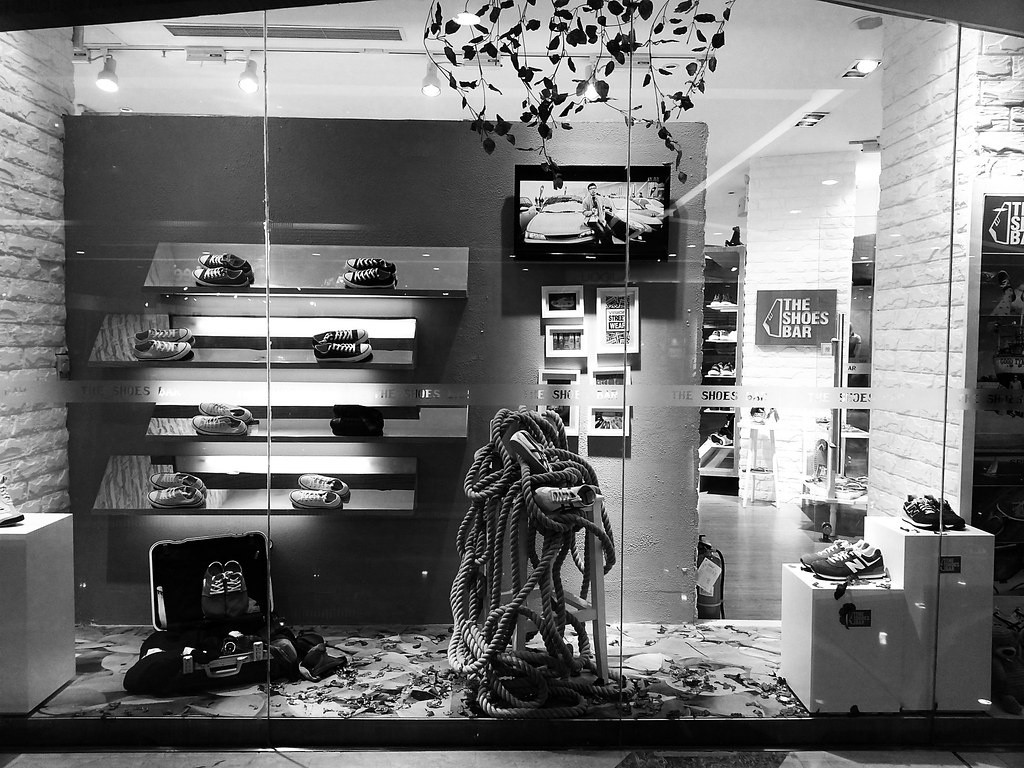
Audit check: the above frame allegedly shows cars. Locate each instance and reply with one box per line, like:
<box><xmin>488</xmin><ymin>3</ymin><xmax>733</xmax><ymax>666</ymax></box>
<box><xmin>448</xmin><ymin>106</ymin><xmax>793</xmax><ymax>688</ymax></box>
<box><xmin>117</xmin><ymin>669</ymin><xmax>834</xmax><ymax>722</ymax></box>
<box><xmin>519</xmin><ymin>193</ymin><xmax>665</xmax><ymax>252</ymax></box>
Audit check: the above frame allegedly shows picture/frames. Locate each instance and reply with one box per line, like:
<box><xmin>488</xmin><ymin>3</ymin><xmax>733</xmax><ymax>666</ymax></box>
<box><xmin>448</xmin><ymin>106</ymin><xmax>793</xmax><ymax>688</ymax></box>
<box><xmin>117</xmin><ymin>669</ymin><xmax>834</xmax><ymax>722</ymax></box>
<box><xmin>546</xmin><ymin>325</ymin><xmax>588</xmax><ymax>357</ymax></box>
<box><xmin>538</xmin><ymin>369</ymin><xmax>580</xmax><ymax>437</ymax></box>
<box><xmin>587</xmin><ymin>366</ymin><xmax>631</xmax><ymax>437</ymax></box>
<box><xmin>596</xmin><ymin>287</ymin><xmax>640</xmax><ymax>354</ymax></box>
<box><xmin>541</xmin><ymin>286</ymin><xmax>584</xmax><ymax>318</ymax></box>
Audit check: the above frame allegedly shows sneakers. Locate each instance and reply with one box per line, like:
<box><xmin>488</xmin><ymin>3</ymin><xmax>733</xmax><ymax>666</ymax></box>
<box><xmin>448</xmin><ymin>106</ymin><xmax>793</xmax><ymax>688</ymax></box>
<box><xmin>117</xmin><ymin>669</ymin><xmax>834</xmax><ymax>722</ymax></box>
<box><xmin>799</xmin><ymin>539</ymin><xmax>869</xmax><ymax>570</ymax></box>
<box><xmin>902</xmin><ymin>495</ymin><xmax>940</xmax><ymax>527</ymax></box>
<box><xmin>708</xmin><ymin>293</ymin><xmax>734</xmax><ymax>445</ymax></box>
<box><xmin>533</xmin><ymin>484</ymin><xmax>596</xmax><ymax>512</ymax></box>
<box><xmin>810</xmin><ymin>548</ymin><xmax>886</xmax><ymax>580</ymax></box>
<box><xmin>197</xmin><ymin>253</ymin><xmax>252</xmax><ymax>275</ymax></box>
<box><xmin>199</xmin><ymin>402</ymin><xmax>252</xmax><ymax>423</ymax></box>
<box><xmin>551</xmin><ymin>296</ymin><xmax>574</xmax><ymax>307</ymax></box>
<box><xmin>0</xmin><ymin>475</ymin><xmax>25</xmax><ymax>527</ymax></box>
<box><xmin>298</xmin><ymin>474</ymin><xmax>349</xmax><ymax>497</ymax></box>
<box><xmin>995</xmin><ymin>500</ymin><xmax>1024</xmax><ymax>520</ymax></box>
<box><xmin>289</xmin><ymin>489</ymin><xmax>341</xmax><ymax>509</ymax></box>
<box><xmin>346</xmin><ymin>258</ymin><xmax>396</xmax><ymax>274</ymax></box>
<box><xmin>134</xmin><ymin>340</ymin><xmax>191</xmax><ymax>360</ymax></box>
<box><xmin>191</xmin><ymin>267</ymin><xmax>249</xmax><ymax>286</ymax></box>
<box><xmin>134</xmin><ymin>327</ymin><xmax>192</xmax><ymax>344</ymax></box>
<box><xmin>925</xmin><ymin>495</ymin><xmax>966</xmax><ymax>528</ymax></box>
<box><xmin>202</xmin><ymin>561</ymin><xmax>224</xmax><ymax>617</ymax></box>
<box><xmin>224</xmin><ymin>560</ymin><xmax>249</xmax><ymax>616</ymax></box>
<box><xmin>314</xmin><ymin>343</ymin><xmax>372</xmax><ymax>361</ymax></box>
<box><xmin>510</xmin><ymin>430</ymin><xmax>553</xmax><ymax>474</ymax></box>
<box><xmin>149</xmin><ymin>472</ymin><xmax>205</xmax><ymax>492</ymax></box>
<box><xmin>312</xmin><ymin>329</ymin><xmax>369</xmax><ymax>348</ymax></box>
<box><xmin>147</xmin><ymin>486</ymin><xmax>204</xmax><ymax>508</ymax></box>
<box><xmin>191</xmin><ymin>415</ymin><xmax>247</xmax><ymax>435</ymax></box>
<box><xmin>342</xmin><ymin>268</ymin><xmax>395</xmax><ymax>288</ymax></box>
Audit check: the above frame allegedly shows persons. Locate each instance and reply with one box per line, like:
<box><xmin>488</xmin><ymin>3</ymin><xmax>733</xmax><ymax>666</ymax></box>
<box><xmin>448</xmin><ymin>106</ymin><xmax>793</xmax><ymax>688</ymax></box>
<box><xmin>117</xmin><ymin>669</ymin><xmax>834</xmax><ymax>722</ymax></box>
<box><xmin>581</xmin><ymin>183</ymin><xmax>616</xmax><ymax>244</ymax></box>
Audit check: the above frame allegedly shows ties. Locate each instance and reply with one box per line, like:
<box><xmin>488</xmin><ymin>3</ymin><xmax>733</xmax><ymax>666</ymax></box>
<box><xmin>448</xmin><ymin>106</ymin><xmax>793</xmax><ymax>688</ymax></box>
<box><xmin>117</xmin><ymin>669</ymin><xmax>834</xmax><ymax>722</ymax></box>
<box><xmin>592</xmin><ymin>196</ymin><xmax>597</xmax><ymax>208</ymax></box>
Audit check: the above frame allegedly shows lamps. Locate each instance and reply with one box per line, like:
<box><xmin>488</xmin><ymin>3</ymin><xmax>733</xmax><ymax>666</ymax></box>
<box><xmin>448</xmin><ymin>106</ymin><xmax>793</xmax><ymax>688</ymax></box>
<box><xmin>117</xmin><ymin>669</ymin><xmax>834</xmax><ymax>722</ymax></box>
<box><xmin>583</xmin><ymin>66</ymin><xmax>599</xmax><ymax>100</ymax></box>
<box><xmin>421</xmin><ymin>63</ymin><xmax>441</xmax><ymax>98</ymax></box>
<box><xmin>95</xmin><ymin>56</ymin><xmax>120</xmax><ymax>94</ymax></box>
<box><xmin>238</xmin><ymin>60</ymin><xmax>258</xmax><ymax>94</ymax></box>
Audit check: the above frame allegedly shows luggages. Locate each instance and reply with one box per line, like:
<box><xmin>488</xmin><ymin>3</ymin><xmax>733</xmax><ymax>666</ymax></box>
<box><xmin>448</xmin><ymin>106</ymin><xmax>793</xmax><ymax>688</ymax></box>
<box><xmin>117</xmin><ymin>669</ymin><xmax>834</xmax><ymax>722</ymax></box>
<box><xmin>149</xmin><ymin>530</ymin><xmax>281</xmax><ymax>691</ymax></box>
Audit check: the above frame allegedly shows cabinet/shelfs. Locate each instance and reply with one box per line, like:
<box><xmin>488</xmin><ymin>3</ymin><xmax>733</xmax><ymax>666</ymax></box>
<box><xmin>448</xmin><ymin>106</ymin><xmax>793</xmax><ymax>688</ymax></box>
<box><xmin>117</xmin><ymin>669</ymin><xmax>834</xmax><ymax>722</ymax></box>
<box><xmin>698</xmin><ymin>245</ymin><xmax>745</xmax><ymax>494</ymax></box>
<box><xmin>799</xmin><ymin>418</ymin><xmax>871</xmax><ymax>540</ymax></box>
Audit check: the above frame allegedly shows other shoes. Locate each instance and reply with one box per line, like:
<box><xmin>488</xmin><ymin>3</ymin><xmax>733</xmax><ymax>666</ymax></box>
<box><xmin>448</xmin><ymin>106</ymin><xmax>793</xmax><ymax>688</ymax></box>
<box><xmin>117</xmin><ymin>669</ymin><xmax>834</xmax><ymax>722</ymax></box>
<box><xmin>332</xmin><ymin>403</ymin><xmax>383</xmax><ymax>418</ymax></box>
<box><xmin>330</xmin><ymin>417</ymin><xmax>384</xmax><ymax>434</ymax></box>
<box><xmin>595</xmin><ymin>417</ymin><xmax>622</xmax><ymax>428</ymax></box>
